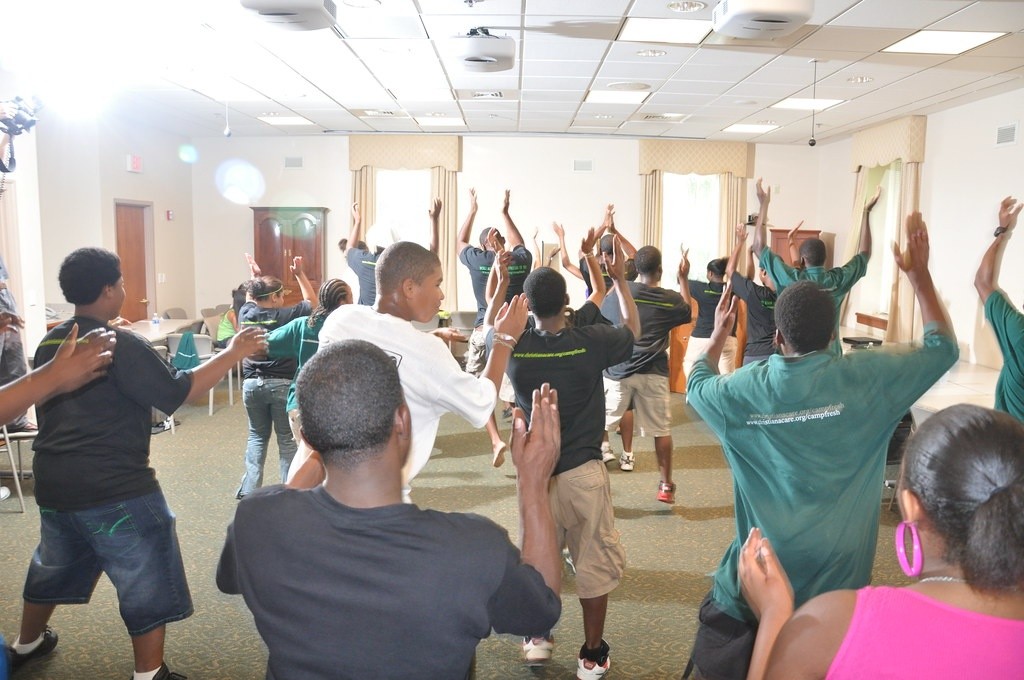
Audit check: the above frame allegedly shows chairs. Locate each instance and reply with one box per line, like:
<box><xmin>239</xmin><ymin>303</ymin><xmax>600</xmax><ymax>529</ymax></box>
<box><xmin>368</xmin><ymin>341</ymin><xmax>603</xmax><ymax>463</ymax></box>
<box><xmin>145</xmin><ymin>305</ymin><xmax>242</xmax><ymax>431</ymax></box>
<box><xmin>0</xmin><ymin>424</ymin><xmax>39</xmax><ymax>514</ymax></box>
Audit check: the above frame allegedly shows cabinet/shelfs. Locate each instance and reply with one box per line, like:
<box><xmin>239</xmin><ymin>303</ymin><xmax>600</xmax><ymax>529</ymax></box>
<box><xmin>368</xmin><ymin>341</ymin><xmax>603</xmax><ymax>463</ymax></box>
<box><xmin>770</xmin><ymin>229</ymin><xmax>822</xmax><ymax>266</ymax></box>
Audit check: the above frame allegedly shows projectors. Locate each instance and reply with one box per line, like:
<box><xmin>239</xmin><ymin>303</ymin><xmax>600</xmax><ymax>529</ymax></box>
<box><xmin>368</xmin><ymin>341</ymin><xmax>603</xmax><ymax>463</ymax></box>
<box><xmin>457</xmin><ymin>36</ymin><xmax>516</xmax><ymax>73</ymax></box>
<box><xmin>241</xmin><ymin>0</ymin><xmax>336</xmax><ymax>31</ymax></box>
<box><xmin>711</xmin><ymin>0</ymin><xmax>815</xmax><ymax>39</ymax></box>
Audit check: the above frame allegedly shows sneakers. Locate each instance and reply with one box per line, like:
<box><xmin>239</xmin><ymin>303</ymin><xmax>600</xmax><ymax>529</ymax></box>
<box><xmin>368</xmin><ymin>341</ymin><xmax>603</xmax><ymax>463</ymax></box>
<box><xmin>600</xmin><ymin>445</ymin><xmax>617</xmax><ymax>463</ymax></box>
<box><xmin>523</xmin><ymin>634</ymin><xmax>554</xmax><ymax>667</ymax></box>
<box><xmin>657</xmin><ymin>480</ymin><xmax>674</xmax><ymax>503</ymax></box>
<box><xmin>9</xmin><ymin>624</ymin><xmax>58</xmax><ymax>673</ymax></box>
<box><xmin>577</xmin><ymin>637</ymin><xmax>610</xmax><ymax>680</ymax></box>
<box><xmin>619</xmin><ymin>449</ymin><xmax>635</xmax><ymax>470</ymax></box>
<box><xmin>130</xmin><ymin>661</ymin><xmax>187</xmax><ymax>680</ymax></box>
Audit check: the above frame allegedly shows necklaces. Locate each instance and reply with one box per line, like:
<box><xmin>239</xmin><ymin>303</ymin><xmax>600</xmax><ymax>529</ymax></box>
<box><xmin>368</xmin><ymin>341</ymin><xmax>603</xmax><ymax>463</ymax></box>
<box><xmin>920</xmin><ymin>576</ymin><xmax>1020</xmax><ymax>590</ymax></box>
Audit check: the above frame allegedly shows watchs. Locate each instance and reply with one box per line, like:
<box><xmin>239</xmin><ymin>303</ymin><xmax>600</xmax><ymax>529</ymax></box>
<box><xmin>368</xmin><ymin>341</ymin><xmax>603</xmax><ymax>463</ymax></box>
<box><xmin>994</xmin><ymin>226</ymin><xmax>1012</xmax><ymax>239</ymax></box>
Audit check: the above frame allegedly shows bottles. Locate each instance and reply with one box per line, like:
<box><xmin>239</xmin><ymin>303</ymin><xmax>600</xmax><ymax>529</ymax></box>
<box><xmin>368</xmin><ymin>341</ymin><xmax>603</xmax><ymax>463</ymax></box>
<box><xmin>245</xmin><ymin>252</ymin><xmax>261</xmax><ymax>273</ymax></box>
<box><xmin>151</xmin><ymin>313</ymin><xmax>159</xmax><ymax>333</ymax></box>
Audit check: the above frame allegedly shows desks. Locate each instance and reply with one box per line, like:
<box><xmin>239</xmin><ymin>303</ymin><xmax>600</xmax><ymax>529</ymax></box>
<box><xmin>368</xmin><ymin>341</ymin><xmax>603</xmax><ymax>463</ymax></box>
<box><xmin>126</xmin><ymin>318</ymin><xmax>197</xmax><ymax>342</ymax></box>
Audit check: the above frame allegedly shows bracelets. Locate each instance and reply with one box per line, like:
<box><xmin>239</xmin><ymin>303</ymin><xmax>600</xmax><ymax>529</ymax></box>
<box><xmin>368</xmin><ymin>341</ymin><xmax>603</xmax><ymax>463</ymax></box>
<box><xmin>492</xmin><ymin>339</ymin><xmax>515</xmax><ymax>351</ymax></box>
<box><xmin>582</xmin><ymin>250</ymin><xmax>594</xmax><ymax>255</ymax></box>
<box><xmin>585</xmin><ymin>255</ymin><xmax>595</xmax><ymax>258</ymax></box>
<box><xmin>493</xmin><ymin>333</ymin><xmax>517</xmax><ymax>346</ymax></box>
<box><xmin>547</xmin><ymin>256</ymin><xmax>553</xmax><ymax>260</ymax></box>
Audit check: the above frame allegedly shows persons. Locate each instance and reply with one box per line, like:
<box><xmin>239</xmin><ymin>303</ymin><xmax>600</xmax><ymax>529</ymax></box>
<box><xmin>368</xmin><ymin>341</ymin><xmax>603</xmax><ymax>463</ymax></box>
<box><xmin>213</xmin><ymin>340</ymin><xmax>562</xmax><ymax>680</ymax></box>
<box><xmin>213</xmin><ymin>176</ymin><xmax>881</xmax><ymax>579</ymax></box>
<box><xmin>974</xmin><ymin>196</ymin><xmax>1024</xmax><ymax>425</ymax></box>
<box><xmin>10</xmin><ymin>247</ymin><xmax>271</xmax><ymax>680</ymax></box>
<box><xmin>682</xmin><ymin>211</ymin><xmax>959</xmax><ymax>680</ymax></box>
<box><xmin>485</xmin><ymin>234</ymin><xmax>641</xmax><ymax>680</ymax></box>
<box><xmin>0</xmin><ymin>254</ymin><xmax>37</xmax><ymax>433</ymax></box>
<box><xmin>0</xmin><ymin>310</ymin><xmax>116</xmax><ymax>680</ymax></box>
<box><xmin>737</xmin><ymin>403</ymin><xmax>1024</xmax><ymax>680</ymax></box>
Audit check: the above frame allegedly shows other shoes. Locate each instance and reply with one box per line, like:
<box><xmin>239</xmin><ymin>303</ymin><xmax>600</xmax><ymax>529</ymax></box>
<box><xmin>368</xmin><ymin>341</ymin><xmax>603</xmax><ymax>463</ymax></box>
<box><xmin>492</xmin><ymin>441</ymin><xmax>507</xmax><ymax>467</ymax></box>
<box><xmin>501</xmin><ymin>406</ymin><xmax>513</xmax><ymax>423</ymax></box>
